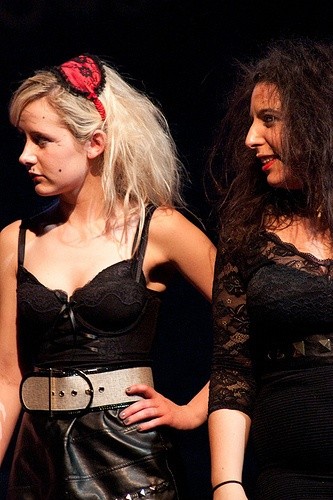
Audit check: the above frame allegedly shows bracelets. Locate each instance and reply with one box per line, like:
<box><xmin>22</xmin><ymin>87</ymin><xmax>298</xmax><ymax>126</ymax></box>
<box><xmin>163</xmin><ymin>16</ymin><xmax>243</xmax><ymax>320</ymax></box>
<box><xmin>211</xmin><ymin>479</ymin><xmax>243</xmax><ymax>494</ymax></box>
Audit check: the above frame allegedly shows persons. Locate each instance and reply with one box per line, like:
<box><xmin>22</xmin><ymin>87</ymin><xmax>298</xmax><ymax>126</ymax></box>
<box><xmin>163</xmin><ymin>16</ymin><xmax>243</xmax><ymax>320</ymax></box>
<box><xmin>0</xmin><ymin>59</ymin><xmax>222</xmax><ymax>500</ymax></box>
<box><xmin>205</xmin><ymin>43</ymin><xmax>333</xmax><ymax>500</ymax></box>
